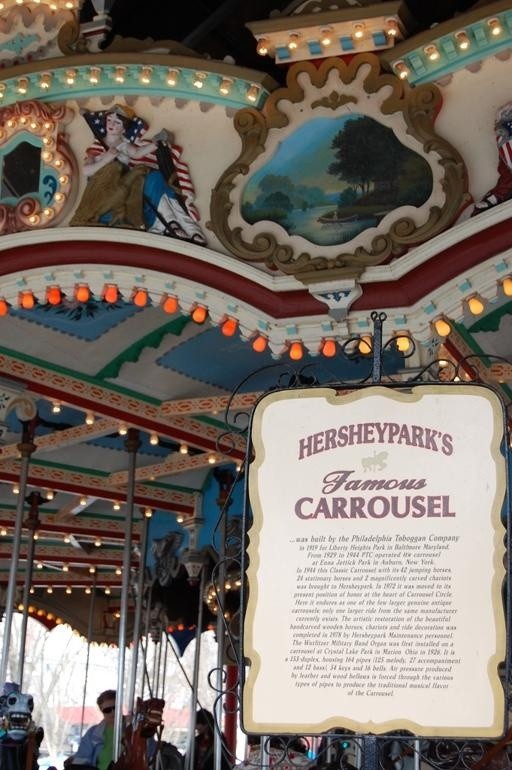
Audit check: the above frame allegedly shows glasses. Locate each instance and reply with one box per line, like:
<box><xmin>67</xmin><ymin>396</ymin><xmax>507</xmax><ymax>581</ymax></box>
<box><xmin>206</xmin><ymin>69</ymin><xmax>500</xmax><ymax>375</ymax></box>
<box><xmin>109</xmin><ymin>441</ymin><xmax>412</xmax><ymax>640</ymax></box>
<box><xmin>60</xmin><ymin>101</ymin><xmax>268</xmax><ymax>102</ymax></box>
<box><xmin>102</xmin><ymin>705</ymin><xmax>115</xmax><ymax>714</ymax></box>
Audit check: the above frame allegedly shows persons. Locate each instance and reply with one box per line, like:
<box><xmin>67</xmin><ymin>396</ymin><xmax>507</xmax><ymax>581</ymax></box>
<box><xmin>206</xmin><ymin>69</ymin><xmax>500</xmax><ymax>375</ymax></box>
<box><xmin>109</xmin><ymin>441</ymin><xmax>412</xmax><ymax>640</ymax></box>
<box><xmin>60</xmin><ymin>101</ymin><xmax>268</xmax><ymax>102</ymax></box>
<box><xmin>70</xmin><ymin>688</ymin><xmax>116</xmax><ymax>770</ymax></box>
<box><xmin>184</xmin><ymin>707</ymin><xmax>235</xmax><ymax>770</ymax></box>
<box><xmin>78</xmin><ymin>100</ymin><xmax>210</xmax><ymax>248</ymax></box>
<box><xmin>230</xmin><ymin>734</ymin><xmax>316</xmax><ymax>770</ymax></box>
<box><xmin>496</xmin><ymin>661</ymin><xmax>512</xmax><ymax>740</ymax></box>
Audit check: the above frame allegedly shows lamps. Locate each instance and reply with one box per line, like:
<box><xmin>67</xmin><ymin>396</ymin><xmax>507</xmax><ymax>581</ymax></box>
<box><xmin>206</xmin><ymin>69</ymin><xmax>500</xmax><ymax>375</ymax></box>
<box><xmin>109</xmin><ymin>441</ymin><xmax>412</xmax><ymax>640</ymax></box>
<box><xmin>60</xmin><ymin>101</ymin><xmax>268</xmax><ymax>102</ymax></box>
<box><xmin>0</xmin><ymin>250</ymin><xmax>512</xmax><ymax>364</ymax></box>
<box><xmin>0</xmin><ymin>114</ymin><xmax>81</xmax><ymax>227</ymax></box>
